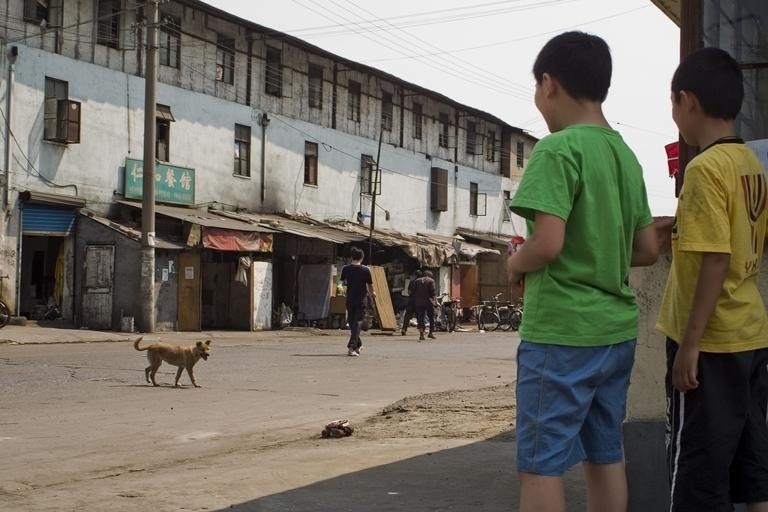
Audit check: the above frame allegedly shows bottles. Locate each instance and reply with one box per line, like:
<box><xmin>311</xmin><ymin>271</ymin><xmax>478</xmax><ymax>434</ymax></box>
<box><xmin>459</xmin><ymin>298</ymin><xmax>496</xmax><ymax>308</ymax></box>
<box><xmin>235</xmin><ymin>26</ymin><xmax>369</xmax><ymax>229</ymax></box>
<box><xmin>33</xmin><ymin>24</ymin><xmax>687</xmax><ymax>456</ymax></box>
<box><xmin>507</xmin><ymin>236</ymin><xmax>526</xmax><ymax>256</ymax></box>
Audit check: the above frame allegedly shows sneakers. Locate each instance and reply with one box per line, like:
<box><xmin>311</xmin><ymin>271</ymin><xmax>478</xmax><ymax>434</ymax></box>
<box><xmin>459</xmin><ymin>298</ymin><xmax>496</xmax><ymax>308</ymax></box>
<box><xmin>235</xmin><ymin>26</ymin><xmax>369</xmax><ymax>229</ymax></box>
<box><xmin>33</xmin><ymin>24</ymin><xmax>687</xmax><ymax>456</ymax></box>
<box><xmin>401</xmin><ymin>330</ymin><xmax>406</xmax><ymax>335</ymax></box>
<box><xmin>428</xmin><ymin>334</ymin><xmax>435</xmax><ymax>339</ymax></box>
<box><xmin>348</xmin><ymin>344</ymin><xmax>363</xmax><ymax>356</ymax></box>
<box><xmin>419</xmin><ymin>335</ymin><xmax>424</xmax><ymax>340</ymax></box>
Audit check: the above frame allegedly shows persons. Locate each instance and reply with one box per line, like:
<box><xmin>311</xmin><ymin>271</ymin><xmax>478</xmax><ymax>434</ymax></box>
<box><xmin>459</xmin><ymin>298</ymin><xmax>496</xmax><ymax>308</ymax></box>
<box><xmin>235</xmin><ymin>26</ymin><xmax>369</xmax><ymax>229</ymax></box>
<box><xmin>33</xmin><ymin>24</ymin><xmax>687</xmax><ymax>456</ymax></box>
<box><xmin>401</xmin><ymin>270</ymin><xmax>428</xmax><ymax>337</ymax></box>
<box><xmin>412</xmin><ymin>269</ymin><xmax>441</xmax><ymax>341</ymax></box>
<box><xmin>656</xmin><ymin>47</ymin><xmax>768</xmax><ymax>512</ymax></box>
<box><xmin>505</xmin><ymin>31</ymin><xmax>660</xmax><ymax>512</ymax></box>
<box><xmin>339</xmin><ymin>248</ymin><xmax>377</xmax><ymax>356</ymax></box>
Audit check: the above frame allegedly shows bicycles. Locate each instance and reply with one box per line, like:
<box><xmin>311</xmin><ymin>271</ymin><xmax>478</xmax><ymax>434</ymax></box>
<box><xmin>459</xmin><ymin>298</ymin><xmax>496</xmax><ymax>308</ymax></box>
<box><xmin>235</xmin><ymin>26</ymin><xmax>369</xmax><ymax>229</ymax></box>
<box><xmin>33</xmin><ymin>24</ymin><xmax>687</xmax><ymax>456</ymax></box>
<box><xmin>478</xmin><ymin>293</ymin><xmax>524</xmax><ymax>332</ymax></box>
<box><xmin>434</xmin><ymin>293</ymin><xmax>461</xmax><ymax>332</ymax></box>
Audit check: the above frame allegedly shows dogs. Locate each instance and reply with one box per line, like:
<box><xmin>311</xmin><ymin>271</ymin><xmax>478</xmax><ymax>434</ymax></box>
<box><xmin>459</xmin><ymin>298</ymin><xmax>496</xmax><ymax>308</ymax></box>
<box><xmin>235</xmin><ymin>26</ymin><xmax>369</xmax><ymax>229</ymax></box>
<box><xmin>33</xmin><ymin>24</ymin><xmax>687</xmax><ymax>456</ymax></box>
<box><xmin>133</xmin><ymin>336</ymin><xmax>212</xmax><ymax>388</ymax></box>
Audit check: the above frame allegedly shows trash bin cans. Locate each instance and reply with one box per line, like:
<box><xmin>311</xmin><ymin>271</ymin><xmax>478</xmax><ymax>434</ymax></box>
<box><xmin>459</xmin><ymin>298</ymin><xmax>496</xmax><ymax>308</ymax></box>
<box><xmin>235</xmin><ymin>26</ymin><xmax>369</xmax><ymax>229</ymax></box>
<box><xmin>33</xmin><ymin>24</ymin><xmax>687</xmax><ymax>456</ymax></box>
<box><xmin>120</xmin><ymin>317</ymin><xmax>135</xmax><ymax>332</ymax></box>
